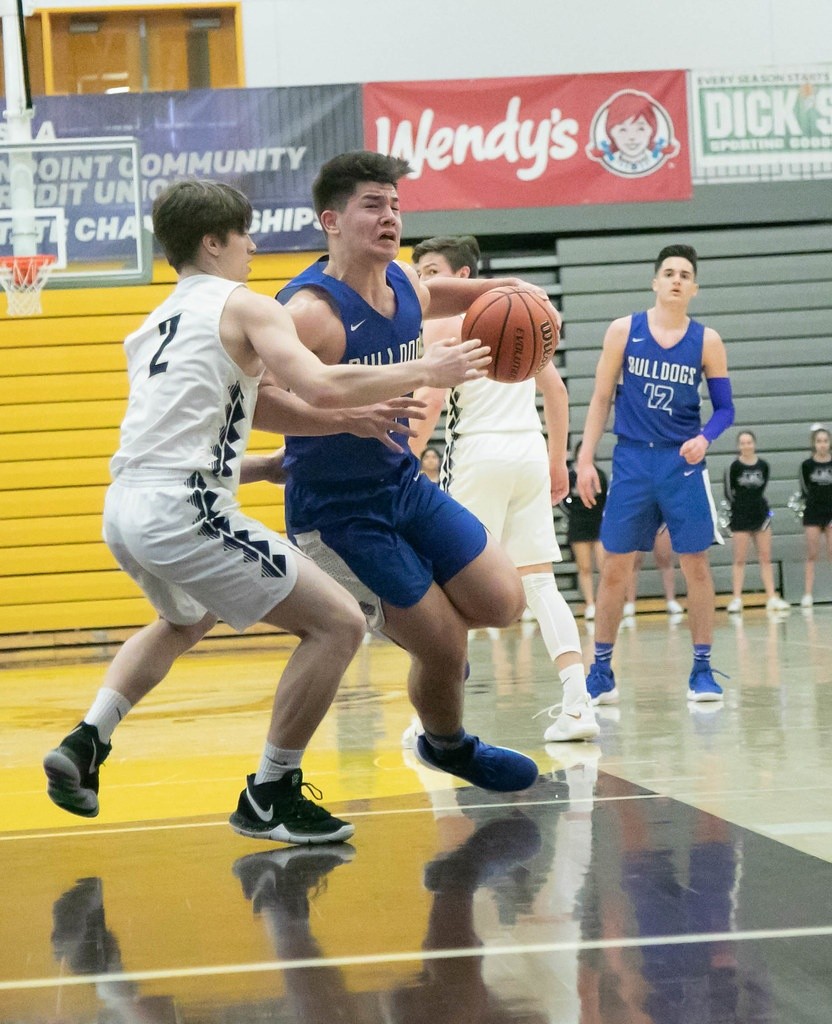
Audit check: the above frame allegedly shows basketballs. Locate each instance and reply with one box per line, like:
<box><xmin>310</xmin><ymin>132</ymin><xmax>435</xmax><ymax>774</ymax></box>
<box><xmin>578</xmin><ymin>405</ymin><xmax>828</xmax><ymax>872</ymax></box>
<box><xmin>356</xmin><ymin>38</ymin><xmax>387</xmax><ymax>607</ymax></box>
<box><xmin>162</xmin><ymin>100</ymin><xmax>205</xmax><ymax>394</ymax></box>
<box><xmin>461</xmin><ymin>285</ymin><xmax>560</xmax><ymax>384</ymax></box>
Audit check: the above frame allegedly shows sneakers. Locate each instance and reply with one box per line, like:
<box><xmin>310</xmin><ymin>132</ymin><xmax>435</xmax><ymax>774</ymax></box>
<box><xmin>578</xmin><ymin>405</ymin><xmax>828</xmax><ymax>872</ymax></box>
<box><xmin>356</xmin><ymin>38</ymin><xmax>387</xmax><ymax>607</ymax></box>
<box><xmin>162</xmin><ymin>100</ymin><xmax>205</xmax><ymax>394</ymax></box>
<box><xmin>685</xmin><ymin>840</ymin><xmax>743</xmax><ymax>923</ymax></box>
<box><xmin>413</xmin><ymin>732</ymin><xmax>540</xmax><ymax>792</ymax></box>
<box><xmin>228</xmin><ymin>768</ymin><xmax>354</xmax><ymax>844</ymax></box>
<box><xmin>520</xmin><ymin>593</ymin><xmax>816</xmax><ymax>618</ymax></box>
<box><xmin>544</xmin><ymin>743</ymin><xmax>602</xmax><ymax>784</ymax></box>
<box><xmin>533</xmin><ymin>692</ymin><xmax>600</xmax><ymax>740</ymax></box>
<box><xmin>687</xmin><ymin>666</ymin><xmax>731</xmax><ymax>700</ymax></box>
<box><xmin>624</xmin><ymin>850</ymin><xmax>681</xmax><ymax>936</ymax></box>
<box><xmin>231</xmin><ymin>843</ymin><xmax>356</xmax><ymax>912</ymax></box>
<box><xmin>51</xmin><ymin>876</ymin><xmax>119</xmax><ymax>970</ymax></box>
<box><xmin>424</xmin><ymin>815</ymin><xmax>542</xmax><ymax>891</ymax></box>
<box><xmin>586</xmin><ymin>664</ymin><xmax>618</xmax><ymax>704</ymax></box>
<box><xmin>43</xmin><ymin>719</ymin><xmax>112</xmax><ymax>818</ymax></box>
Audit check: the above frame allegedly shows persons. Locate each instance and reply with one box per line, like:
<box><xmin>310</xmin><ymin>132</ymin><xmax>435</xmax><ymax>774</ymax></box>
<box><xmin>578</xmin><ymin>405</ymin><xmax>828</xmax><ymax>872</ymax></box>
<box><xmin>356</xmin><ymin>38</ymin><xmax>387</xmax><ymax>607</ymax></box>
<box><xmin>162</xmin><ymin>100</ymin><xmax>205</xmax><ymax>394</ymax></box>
<box><xmin>724</xmin><ymin>431</ymin><xmax>790</xmax><ymax>611</ymax></box>
<box><xmin>801</xmin><ymin>428</ymin><xmax>832</xmax><ymax>607</ymax></box>
<box><xmin>45</xmin><ymin>181</ymin><xmax>492</xmax><ymax>842</ymax></box>
<box><xmin>577</xmin><ymin>244</ymin><xmax>735</xmax><ymax>701</ymax></box>
<box><xmin>560</xmin><ymin>442</ymin><xmax>609</xmax><ymax>618</ymax></box>
<box><xmin>252</xmin><ymin>152</ymin><xmax>562</xmax><ymax>793</ymax></box>
<box><xmin>624</xmin><ymin>528</ymin><xmax>684</xmax><ymax>613</ymax></box>
<box><xmin>422</xmin><ymin>448</ymin><xmax>442</xmax><ymax>483</ymax></box>
<box><xmin>409</xmin><ymin>236</ymin><xmax>600</xmax><ymax>738</ymax></box>
